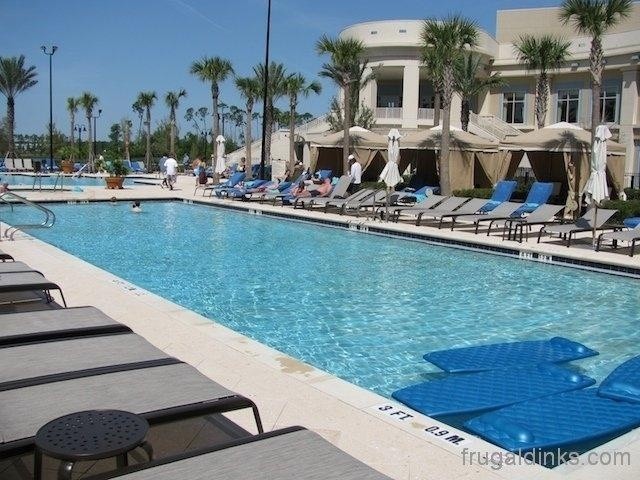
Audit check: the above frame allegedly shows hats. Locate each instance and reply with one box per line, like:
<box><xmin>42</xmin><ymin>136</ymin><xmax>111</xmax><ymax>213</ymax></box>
<box><xmin>347</xmin><ymin>155</ymin><xmax>355</xmax><ymax>163</ymax></box>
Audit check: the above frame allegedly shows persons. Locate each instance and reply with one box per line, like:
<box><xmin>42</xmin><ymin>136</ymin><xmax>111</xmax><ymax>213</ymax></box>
<box><xmin>198</xmin><ymin>155</ymin><xmax>207</xmax><ymax>176</ymax></box>
<box><xmin>157</xmin><ymin>151</ymin><xmax>169</xmax><ymax>189</ymax></box>
<box><xmin>235</xmin><ymin>157</ymin><xmax>246</xmax><ymax>173</ymax></box>
<box><xmin>182</xmin><ymin>152</ymin><xmax>190</xmax><ymax>177</ymax></box>
<box><xmin>289</xmin><ymin>176</ymin><xmax>335</xmax><ymax>199</ymax></box>
<box><xmin>129</xmin><ymin>200</ymin><xmax>143</xmax><ymax>212</ymax></box>
<box><xmin>345</xmin><ymin>155</ymin><xmax>363</xmax><ymax>197</ymax></box>
<box><xmin>191</xmin><ymin>152</ymin><xmax>203</xmax><ymax>187</ymax></box>
<box><xmin>237</xmin><ymin>178</ymin><xmax>283</xmax><ymax>193</ymax></box>
<box><xmin>110</xmin><ymin>196</ymin><xmax>118</xmax><ymax>204</ymax></box>
<box><xmin>163</xmin><ymin>152</ymin><xmax>180</xmax><ymax>191</ymax></box>
<box><xmin>95</xmin><ymin>152</ymin><xmax>108</xmax><ymax>175</ymax></box>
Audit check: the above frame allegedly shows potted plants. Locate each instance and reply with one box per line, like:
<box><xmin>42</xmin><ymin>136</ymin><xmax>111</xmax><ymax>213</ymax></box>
<box><xmin>93</xmin><ymin>150</ymin><xmax>131</xmax><ymax>188</ymax></box>
<box><xmin>56</xmin><ymin>144</ymin><xmax>81</xmax><ymax>174</ymax></box>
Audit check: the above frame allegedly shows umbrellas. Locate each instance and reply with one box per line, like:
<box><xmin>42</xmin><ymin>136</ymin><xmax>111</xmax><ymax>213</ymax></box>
<box><xmin>215</xmin><ymin>133</ymin><xmax>227</xmax><ymax>183</ymax></box>
<box><xmin>580</xmin><ymin>123</ymin><xmax>614</xmax><ymax>249</ymax></box>
<box><xmin>377</xmin><ymin>127</ymin><xmax>403</xmax><ymax>220</ymax></box>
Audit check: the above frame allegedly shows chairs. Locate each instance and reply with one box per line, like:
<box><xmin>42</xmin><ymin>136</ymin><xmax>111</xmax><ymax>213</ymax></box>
<box><xmin>92</xmin><ymin>425</ymin><xmax>395</xmax><ymax>480</ymax></box>
<box><xmin>0</xmin><ymin>248</ymin><xmax>264</xmax><ymax>479</ymax></box>
<box><xmin>0</xmin><ymin>157</ymin><xmax>60</xmax><ymax>174</ymax></box>
<box><xmin>377</xmin><ymin>181</ymin><xmax>640</xmax><ymax>258</ymax></box>
<box><xmin>203</xmin><ymin>165</ymin><xmax>381</xmax><ymax>219</ymax></box>
<box><xmin>123</xmin><ymin>159</ymin><xmax>147</xmax><ymax>174</ymax></box>
